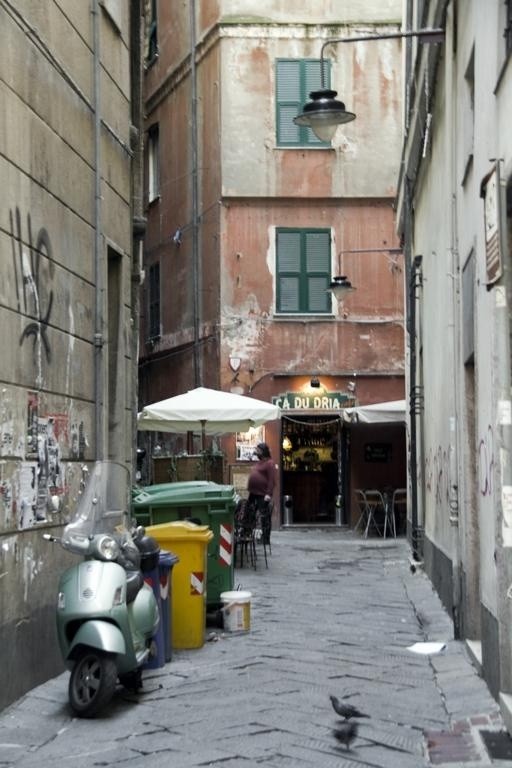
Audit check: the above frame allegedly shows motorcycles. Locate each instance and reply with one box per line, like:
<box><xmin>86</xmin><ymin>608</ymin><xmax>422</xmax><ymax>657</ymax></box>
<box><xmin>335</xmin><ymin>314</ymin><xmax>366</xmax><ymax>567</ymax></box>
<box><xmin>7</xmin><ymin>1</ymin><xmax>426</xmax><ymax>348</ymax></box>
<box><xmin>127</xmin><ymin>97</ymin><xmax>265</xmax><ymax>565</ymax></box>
<box><xmin>42</xmin><ymin>523</ymin><xmax>162</xmax><ymax>718</ymax></box>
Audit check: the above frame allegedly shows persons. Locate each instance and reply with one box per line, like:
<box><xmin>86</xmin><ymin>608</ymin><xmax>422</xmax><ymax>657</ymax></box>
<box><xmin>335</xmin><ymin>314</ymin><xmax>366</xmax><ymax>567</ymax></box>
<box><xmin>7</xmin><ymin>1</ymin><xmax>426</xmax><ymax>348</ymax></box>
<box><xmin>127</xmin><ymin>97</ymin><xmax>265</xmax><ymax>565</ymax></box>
<box><xmin>315</xmin><ymin>461</ymin><xmax>337</xmax><ymax>516</ymax></box>
<box><xmin>240</xmin><ymin>443</ymin><xmax>277</xmax><ymax>544</ymax></box>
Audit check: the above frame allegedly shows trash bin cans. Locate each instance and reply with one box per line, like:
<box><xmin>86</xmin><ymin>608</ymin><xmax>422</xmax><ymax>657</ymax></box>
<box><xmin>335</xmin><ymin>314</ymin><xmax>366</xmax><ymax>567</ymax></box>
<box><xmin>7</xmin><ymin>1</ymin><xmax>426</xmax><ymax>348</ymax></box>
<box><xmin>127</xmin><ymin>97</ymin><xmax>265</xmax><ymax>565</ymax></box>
<box><xmin>131</xmin><ymin>481</ymin><xmax>242</xmax><ymax>669</ymax></box>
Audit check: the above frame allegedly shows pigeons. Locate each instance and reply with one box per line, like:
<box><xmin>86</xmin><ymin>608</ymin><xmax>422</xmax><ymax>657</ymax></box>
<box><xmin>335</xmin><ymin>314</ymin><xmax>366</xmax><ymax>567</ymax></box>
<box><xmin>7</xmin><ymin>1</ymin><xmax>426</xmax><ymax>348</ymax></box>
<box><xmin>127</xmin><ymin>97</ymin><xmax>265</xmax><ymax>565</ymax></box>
<box><xmin>332</xmin><ymin>721</ymin><xmax>361</xmax><ymax>750</ymax></box>
<box><xmin>330</xmin><ymin>695</ymin><xmax>370</xmax><ymax>723</ymax></box>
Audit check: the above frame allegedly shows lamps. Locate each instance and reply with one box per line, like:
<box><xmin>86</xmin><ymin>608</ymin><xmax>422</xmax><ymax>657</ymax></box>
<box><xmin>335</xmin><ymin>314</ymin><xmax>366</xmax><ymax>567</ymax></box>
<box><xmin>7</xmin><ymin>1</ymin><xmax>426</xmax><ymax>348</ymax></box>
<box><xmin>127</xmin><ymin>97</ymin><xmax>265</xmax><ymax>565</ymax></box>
<box><xmin>292</xmin><ymin>23</ymin><xmax>445</xmax><ymax>146</ymax></box>
<box><xmin>310</xmin><ymin>373</ymin><xmax>320</xmax><ymax>388</ymax></box>
<box><xmin>321</xmin><ymin>247</ymin><xmax>403</xmax><ymax>302</ymax></box>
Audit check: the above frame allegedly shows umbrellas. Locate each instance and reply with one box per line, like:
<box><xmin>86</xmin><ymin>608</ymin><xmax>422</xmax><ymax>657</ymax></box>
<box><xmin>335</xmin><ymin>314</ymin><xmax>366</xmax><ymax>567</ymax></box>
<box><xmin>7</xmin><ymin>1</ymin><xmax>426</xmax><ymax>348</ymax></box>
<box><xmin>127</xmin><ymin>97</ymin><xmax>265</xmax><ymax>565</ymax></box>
<box><xmin>138</xmin><ymin>386</ymin><xmax>281</xmax><ymax>480</ymax></box>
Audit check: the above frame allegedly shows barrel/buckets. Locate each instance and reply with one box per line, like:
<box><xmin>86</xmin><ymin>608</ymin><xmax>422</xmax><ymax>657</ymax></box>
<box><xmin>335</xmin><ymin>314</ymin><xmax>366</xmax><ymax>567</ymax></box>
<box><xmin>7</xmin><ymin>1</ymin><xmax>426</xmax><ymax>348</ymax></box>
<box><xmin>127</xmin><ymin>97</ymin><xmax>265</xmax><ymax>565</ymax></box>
<box><xmin>220</xmin><ymin>590</ymin><xmax>251</xmax><ymax>632</ymax></box>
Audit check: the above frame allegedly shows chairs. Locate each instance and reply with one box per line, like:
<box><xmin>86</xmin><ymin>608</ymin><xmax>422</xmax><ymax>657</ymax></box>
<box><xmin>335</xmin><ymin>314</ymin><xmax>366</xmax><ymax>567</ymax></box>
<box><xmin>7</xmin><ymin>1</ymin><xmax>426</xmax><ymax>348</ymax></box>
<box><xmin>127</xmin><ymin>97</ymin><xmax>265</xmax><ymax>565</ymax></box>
<box><xmin>351</xmin><ymin>484</ymin><xmax>406</xmax><ymax>543</ymax></box>
<box><xmin>232</xmin><ymin>493</ymin><xmax>269</xmax><ymax>572</ymax></box>
